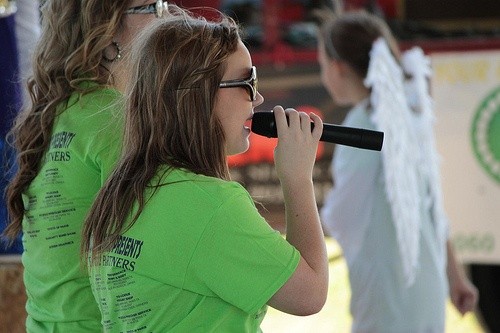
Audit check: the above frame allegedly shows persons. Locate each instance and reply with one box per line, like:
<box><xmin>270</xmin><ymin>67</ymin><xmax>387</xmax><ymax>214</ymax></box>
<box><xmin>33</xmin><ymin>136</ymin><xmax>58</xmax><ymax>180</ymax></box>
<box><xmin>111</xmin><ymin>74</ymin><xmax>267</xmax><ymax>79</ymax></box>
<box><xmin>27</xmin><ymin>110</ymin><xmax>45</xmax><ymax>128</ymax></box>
<box><xmin>318</xmin><ymin>11</ymin><xmax>480</xmax><ymax>333</ymax></box>
<box><xmin>79</xmin><ymin>17</ymin><xmax>330</xmax><ymax>333</ymax></box>
<box><xmin>5</xmin><ymin>0</ymin><xmax>179</xmax><ymax>333</ymax></box>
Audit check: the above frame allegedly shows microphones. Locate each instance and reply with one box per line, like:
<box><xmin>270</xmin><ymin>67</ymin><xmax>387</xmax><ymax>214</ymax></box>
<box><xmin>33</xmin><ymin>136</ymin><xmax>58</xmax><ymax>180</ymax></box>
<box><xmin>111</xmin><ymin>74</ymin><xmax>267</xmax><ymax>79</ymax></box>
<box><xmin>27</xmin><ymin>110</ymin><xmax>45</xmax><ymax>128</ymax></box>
<box><xmin>251</xmin><ymin>111</ymin><xmax>384</xmax><ymax>152</ymax></box>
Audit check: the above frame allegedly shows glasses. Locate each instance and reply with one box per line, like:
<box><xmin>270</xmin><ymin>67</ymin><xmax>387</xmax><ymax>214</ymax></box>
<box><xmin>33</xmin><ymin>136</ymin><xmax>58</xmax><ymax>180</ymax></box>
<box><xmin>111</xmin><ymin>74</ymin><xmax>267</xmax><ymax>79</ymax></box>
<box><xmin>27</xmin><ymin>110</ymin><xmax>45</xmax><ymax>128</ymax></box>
<box><xmin>220</xmin><ymin>66</ymin><xmax>257</xmax><ymax>101</ymax></box>
<box><xmin>121</xmin><ymin>0</ymin><xmax>168</xmax><ymax>17</ymax></box>
